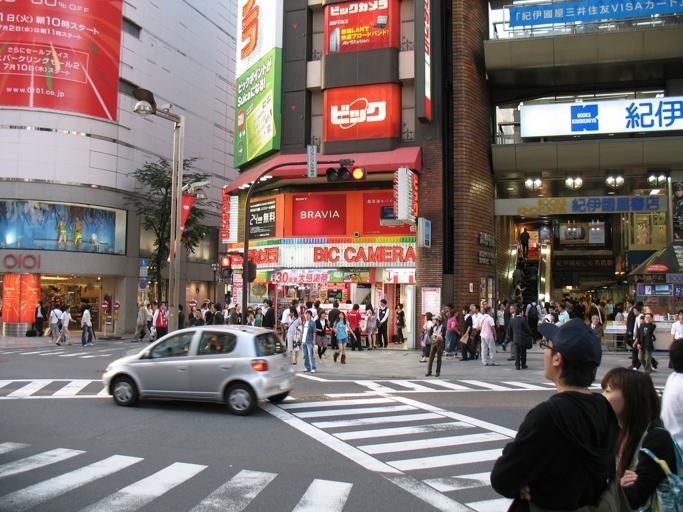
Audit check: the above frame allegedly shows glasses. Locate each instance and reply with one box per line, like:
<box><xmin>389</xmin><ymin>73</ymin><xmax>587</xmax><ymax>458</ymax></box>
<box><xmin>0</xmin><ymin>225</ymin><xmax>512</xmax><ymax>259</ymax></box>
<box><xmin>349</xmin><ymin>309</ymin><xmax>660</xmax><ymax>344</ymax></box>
<box><xmin>539</xmin><ymin>339</ymin><xmax>556</xmax><ymax>354</ymax></box>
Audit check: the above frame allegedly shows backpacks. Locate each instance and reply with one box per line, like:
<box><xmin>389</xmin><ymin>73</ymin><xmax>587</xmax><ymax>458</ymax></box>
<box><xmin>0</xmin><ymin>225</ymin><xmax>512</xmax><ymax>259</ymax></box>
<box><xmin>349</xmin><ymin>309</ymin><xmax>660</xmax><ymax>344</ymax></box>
<box><xmin>57</xmin><ymin>319</ymin><xmax>62</xmax><ymax>330</ymax></box>
<box><xmin>528</xmin><ymin>306</ymin><xmax>538</xmax><ymax>322</ymax></box>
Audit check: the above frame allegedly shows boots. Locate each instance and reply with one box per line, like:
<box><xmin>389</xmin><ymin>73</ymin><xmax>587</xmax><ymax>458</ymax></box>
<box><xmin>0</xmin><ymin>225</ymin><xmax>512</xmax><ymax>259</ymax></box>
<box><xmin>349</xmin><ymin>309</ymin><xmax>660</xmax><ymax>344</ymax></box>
<box><xmin>334</xmin><ymin>352</ymin><xmax>339</xmax><ymax>362</ymax></box>
<box><xmin>341</xmin><ymin>354</ymin><xmax>346</xmax><ymax>364</ymax></box>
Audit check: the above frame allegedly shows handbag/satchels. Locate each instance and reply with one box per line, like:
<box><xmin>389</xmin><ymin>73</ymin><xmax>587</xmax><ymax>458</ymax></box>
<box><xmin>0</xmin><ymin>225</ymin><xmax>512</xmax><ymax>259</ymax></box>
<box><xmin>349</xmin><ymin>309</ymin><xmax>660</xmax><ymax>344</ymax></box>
<box><xmin>632</xmin><ymin>446</ymin><xmax>682</xmax><ymax>511</ymax></box>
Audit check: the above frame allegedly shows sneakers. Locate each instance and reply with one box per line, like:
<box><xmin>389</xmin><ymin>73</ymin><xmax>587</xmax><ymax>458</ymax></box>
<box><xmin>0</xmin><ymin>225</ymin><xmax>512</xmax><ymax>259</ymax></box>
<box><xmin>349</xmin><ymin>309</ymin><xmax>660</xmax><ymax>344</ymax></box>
<box><xmin>482</xmin><ymin>361</ymin><xmax>499</xmax><ymax>366</ymax></box>
<box><xmin>419</xmin><ymin>358</ymin><xmax>428</xmax><ymax>363</ymax></box>
<box><xmin>515</xmin><ymin>365</ymin><xmax>520</xmax><ymax>370</ymax></box>
<box><xmin>350</xmin><ymin>344</ymin><xmax>387</xmax><ymax>351</ymax></box>
<box><xmin>311</xmin><ymin>369</ymin><xmax>317</xmax><ymax>373</ymax></box>
<box><xmin>320</xmin><ymin>354</ymin><xmax>325</xmax><ymax>364</ymax></box>
<box><xmin>435</xmin><ymin>372</ymin><xmax>439</xmax><ymax>377</ymax></box>
<box><xmin>82</xmin><ymin>341</ymin><xmax>94</xmax><ymax>347</ymax></box>
<box><xmin>522</xmin><ymin>365</ymin><xmax>529</xmax><ymax>369</ymax></box>
<box><xmin>304</xmin><ymin>368</ymin><xmax>310</xmax><ymax>372</ymax></box>
<box><xmin>426</xmin><ymin>372</ymin><xmax>431</xmax><ymax>376</ymax></box>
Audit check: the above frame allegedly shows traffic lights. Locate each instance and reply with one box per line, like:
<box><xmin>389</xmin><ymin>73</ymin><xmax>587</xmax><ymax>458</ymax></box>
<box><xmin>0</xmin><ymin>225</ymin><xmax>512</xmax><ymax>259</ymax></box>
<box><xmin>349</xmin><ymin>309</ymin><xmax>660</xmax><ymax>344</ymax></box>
<box><xmin>325</xmin><ymin>166</ymin><xmax>367</xmax><ymax>183</ymax></box>
<box><xmin>220</xmin><ymin>255</ymin><xmax>233</xmax><ymax>280</ymax></box>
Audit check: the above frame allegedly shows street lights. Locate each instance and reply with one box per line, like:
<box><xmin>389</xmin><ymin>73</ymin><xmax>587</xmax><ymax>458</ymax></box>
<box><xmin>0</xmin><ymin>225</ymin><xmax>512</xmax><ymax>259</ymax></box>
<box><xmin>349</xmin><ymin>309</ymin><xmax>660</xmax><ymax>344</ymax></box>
<box><xmin>209</xmin><ymin>264</ymin><xmax>221</xmax><ymax>304</ymax></box>
<box><xmin>97</xmin><ymin>275</ymin><xmax>104</xmax><ymax>330</ymax></box>
<box><xmin>129</xmin><ymin>87</ymin><xmax>183</xmax><ymax>337</ymax></box>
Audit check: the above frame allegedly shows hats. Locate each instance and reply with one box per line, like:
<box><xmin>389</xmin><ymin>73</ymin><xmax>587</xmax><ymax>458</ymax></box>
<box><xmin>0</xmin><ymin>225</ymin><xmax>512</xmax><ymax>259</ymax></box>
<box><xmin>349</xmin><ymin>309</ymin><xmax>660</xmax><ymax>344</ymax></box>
<box><xmin>431</xmin><ymin>314</ymin><xmax>443</xmax><ymax>322</ymax></box>
<box><xmin>542</xmin><ymin>318</ymin><xmax>602</xmax><ymax>370</ymax></box>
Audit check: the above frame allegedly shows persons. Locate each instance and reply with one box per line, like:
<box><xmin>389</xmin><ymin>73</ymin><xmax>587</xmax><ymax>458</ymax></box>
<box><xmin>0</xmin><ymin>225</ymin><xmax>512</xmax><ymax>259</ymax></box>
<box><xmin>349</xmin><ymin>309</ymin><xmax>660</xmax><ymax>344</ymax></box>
<box><xmin>417</xmin><ymin>293</ymin><xmax>682</xmax><ymax>378</ymax></box>
<box><xmin>510</xmin><ymin>265</ymin><xmax>525</xmax><ymax>286</ymax></box>
<box><xmin>33</xmin><ymin>299</ymin><xmax>46</xmax><ymax>337</ymax></box>
<box><xmin>595</xmin><ymin>365</ymin><xmax>677</xmax><ymax>512</ymax></box>
<box><xmin>72</xmin><ymin>216</ymin><xmax>82</xmax><ymax>249</ymax></box>
<box><xmin>519</xmin><ymin>228</ymin><xmax>530</xmax><ymax>259</ymax></box>
<box><xmin>393</xmin><ymin>304</ymin><xmax>406</xmax><ymax>344</ymax></box>
<box><xmin>489</xmin><ymin>315</ymin><xmax>618</xmax><ymax>511</ymax></box>
<box><xmin>510</xmin><ymin>280</ymin><xmax>526</xmax><ymax>303</ymax></box>
<box><xmin>128</xmin><ymin>297</ymin><xmax>388</xmax><ymax>374</ymax></box>
<box><xmin>48</xmin><ymin>304</ymin><xmax>94</xmax><ymax>347</ymax></box>
<box><xmin>660</xmin><ymin>336</ymin><xmax>682</xmax><ymax>450</ymax></box>
<box><xmin>55</xmin><ymin>216</ymin><xmax>69</xmax><ymax>250</ymax></box>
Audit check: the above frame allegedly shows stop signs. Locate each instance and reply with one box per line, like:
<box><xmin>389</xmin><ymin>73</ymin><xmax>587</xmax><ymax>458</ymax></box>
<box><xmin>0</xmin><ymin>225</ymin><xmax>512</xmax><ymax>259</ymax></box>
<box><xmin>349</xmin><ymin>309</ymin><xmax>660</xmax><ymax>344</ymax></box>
<box><xmin>101</xmin><ymin>302</ymin><xmax>107</xmax><ymax>310</ymax></box>
<box><xmin>113</xmin><ymin>302</ymin><xmax>120</xmax><ymax>309</ymax></box>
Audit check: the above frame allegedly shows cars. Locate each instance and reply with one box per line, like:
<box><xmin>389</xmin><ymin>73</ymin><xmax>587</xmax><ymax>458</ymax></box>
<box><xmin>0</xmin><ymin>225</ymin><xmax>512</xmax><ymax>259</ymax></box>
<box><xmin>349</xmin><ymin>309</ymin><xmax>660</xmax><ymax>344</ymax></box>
<box><xmin>101</xmin><ymin>323</ymin><xmax>296</xmax><ymax>416</ymax></box>
